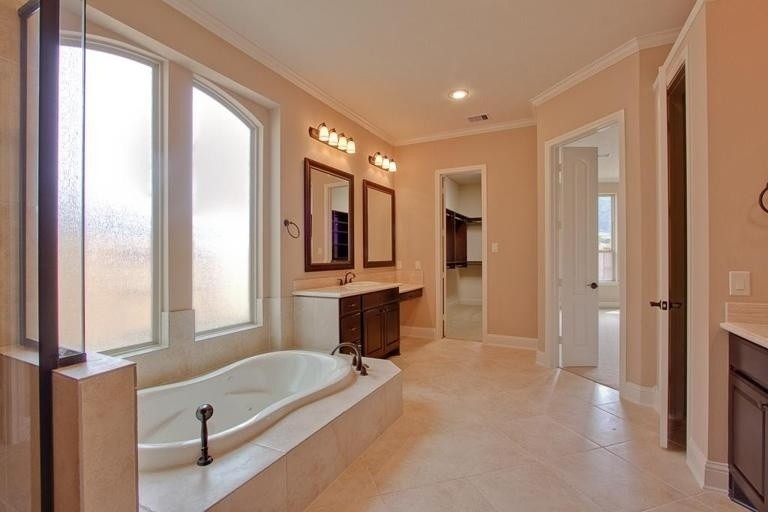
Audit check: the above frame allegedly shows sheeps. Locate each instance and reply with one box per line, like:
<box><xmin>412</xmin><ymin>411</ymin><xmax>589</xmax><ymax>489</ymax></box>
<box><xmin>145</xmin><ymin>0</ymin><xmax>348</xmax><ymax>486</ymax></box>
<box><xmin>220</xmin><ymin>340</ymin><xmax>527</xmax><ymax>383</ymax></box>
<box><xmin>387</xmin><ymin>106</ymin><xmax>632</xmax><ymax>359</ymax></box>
<box><xmin>331</xmin><ymin>341</ymin><xmax>363</xmax><ymax>369</ymax></box>
<box><xmin>344</xmin><ymin>271</ymin><xmax>356</xmax><ymax>282</ymax></box>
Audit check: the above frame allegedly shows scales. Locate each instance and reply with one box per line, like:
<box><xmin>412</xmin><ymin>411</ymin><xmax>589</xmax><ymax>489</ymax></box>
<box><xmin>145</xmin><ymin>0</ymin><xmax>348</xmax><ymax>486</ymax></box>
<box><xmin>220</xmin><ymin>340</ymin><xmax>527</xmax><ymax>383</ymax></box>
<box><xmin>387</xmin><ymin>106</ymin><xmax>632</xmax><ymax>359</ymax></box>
<box><xmin>137</xmin><ymin>348</ymin><xmax>356</xmax><ymax>470</ymax></box>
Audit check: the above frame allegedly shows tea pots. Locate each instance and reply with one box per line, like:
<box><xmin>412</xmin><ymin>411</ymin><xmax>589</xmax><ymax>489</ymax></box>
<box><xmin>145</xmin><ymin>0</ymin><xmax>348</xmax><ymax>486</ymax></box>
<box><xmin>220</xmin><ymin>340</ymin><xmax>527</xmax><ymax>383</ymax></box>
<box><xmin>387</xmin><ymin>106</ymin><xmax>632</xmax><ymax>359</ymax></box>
<box><xmin>304</xmin><ymin>157</ymin><xmax>354</xmax><ymax>272</ymax></box>
<box><xmin>363</xmin><ymin>179</ymin><xmax>395</xmax><ymax>268</ymax></box>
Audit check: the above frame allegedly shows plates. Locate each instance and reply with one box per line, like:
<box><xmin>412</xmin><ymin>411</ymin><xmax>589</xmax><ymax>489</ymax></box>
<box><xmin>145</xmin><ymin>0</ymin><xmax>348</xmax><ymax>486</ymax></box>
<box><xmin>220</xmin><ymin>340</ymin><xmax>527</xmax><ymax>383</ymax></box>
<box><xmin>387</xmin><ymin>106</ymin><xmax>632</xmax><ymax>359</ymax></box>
<box><xmin>332</xmin><ymin>210</ymin><xmax>348</xmax><ymax>260</ymax></box>
<box><xmin>446</xmin><ymin>208</ymin><xmax>467</xmax><ymax>269</ymax></box>
<box><xmin>728</xmin><ymin>332</ymin><xmax>768</xmax><ymax>512</ymax></box>
<box><xmin>293</xmin><ymin>287</ymin><xmax>400</xmax><ymax>358</ymax></box>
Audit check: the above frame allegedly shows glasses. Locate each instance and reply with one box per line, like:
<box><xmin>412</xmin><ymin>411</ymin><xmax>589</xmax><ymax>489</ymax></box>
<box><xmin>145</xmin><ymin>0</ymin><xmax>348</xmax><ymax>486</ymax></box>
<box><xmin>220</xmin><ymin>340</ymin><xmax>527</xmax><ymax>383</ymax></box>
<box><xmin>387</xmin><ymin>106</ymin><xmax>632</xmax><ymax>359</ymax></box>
<box><xmin>309</xmin><ymin>123</ymin><xmax>356</xmax><ymax>154</ymax></box>
<box><xmin>368</xmin><ymin>152</ymin><xmax>397</xmax><ymax>172</ymax></box>
